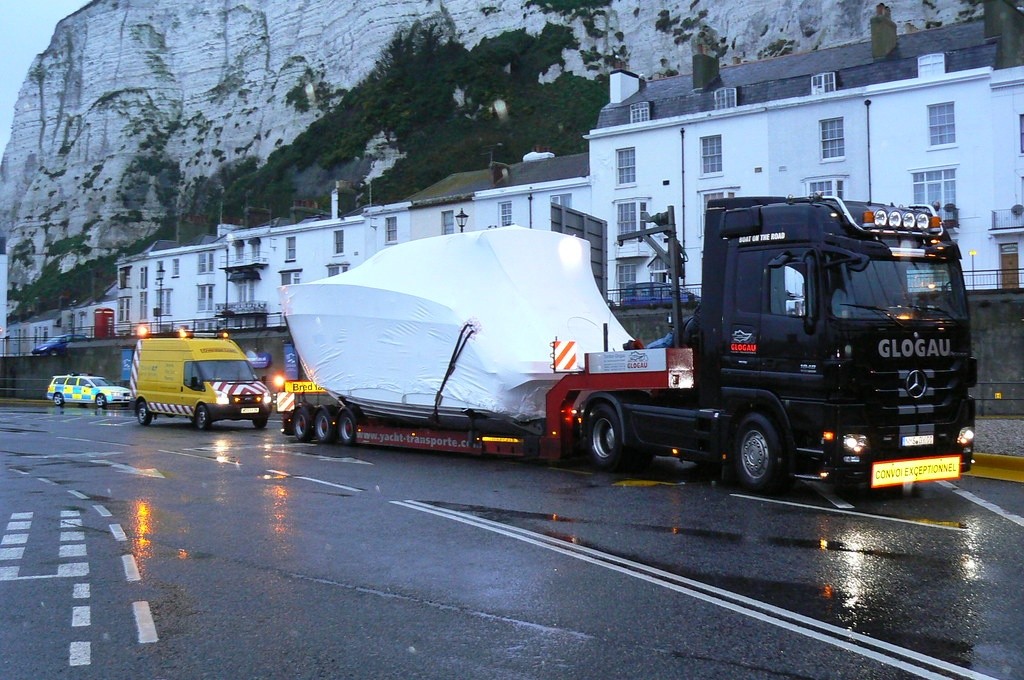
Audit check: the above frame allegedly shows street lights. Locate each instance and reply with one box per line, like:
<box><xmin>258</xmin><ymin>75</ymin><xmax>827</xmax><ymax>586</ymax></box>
<box><xmin>154</xmin><ymin>261</ymin><xmax>167</xmax><ymax>336</ymax></box>
<box><xmin>222</xmin><ymin>234</ymin><xmax>232</xmax><ymax>329</ymax></box>
<box><xmin>970</xmin><ymin>251</ymin><xmax>976</xmax><ymax>290</ymax></box>
<box><xmin>455</xmin><ymin>209</ymin><xmax>469</xmax><ymax>233</ymax></box>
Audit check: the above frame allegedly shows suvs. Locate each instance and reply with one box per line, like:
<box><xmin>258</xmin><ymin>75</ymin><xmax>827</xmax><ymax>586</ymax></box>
<box><xmin>619</xmin><ymin>279</ymin><xmax>702</xmax><ymax>310</ymax></box>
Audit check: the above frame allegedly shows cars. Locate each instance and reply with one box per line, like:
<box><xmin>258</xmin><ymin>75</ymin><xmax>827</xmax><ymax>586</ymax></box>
<box><xmin>32</xmin><ymin>333</ymin><xmax>90</xmax><ymax>359</ymax></box>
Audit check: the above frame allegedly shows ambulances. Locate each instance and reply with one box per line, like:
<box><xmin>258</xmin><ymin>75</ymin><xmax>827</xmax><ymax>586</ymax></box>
<box><xmin>46</xmin><ymin>373</ymin><xmax>131</xmax><ymax>407</ymax></box>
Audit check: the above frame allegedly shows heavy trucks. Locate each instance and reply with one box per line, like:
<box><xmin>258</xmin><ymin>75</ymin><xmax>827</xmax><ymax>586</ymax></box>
<box><xmin>276</xmin><ymin>194</ymin><xmax>978</xmax><ymax>498</ymax></box>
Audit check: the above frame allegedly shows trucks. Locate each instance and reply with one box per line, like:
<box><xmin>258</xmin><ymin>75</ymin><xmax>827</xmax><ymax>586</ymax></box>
<box><xmin>130</xmin><ymin>330</ymin><xmax>273</xmax><ymax>432</ymax></box>
